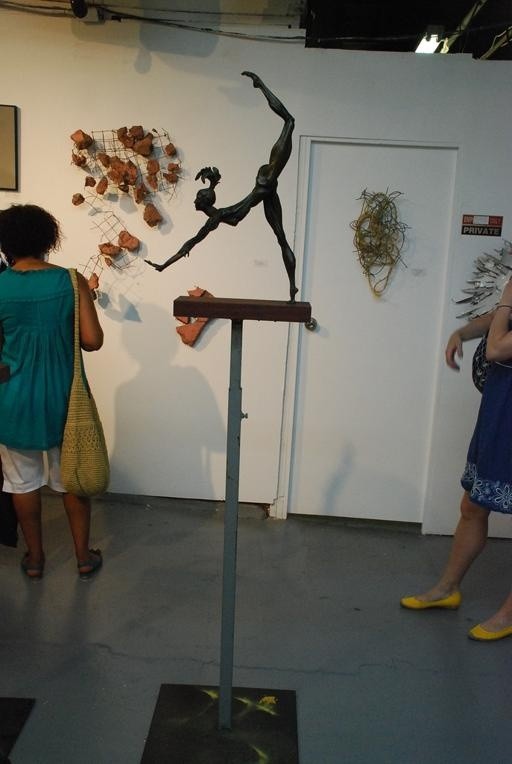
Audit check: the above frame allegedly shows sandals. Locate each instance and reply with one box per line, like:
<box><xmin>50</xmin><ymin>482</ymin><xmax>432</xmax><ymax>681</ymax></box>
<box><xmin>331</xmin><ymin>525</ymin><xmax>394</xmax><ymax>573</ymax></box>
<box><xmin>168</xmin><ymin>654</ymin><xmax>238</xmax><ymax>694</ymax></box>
<box><xmin>20</xmin><ymin>553</ymin><xmax>46</xmax><ymax>585</ymax></box>
<box><xmin>77</xmin><ymin>549</ymin><xmax>103</xmax><ymax>583</ymax></box>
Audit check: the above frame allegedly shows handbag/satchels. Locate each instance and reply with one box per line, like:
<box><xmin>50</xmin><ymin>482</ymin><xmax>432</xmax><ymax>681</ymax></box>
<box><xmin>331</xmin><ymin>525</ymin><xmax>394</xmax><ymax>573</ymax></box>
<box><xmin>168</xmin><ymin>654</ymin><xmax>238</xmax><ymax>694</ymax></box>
<box><xmin>60</xmin><ymin>375</ymin><xmax>110</xmax><ymax>499</ymax></box>
<box><xmin>470</xmin><ymin>331</ymin><xmax>494</xmax><ymax>396</ymax></box>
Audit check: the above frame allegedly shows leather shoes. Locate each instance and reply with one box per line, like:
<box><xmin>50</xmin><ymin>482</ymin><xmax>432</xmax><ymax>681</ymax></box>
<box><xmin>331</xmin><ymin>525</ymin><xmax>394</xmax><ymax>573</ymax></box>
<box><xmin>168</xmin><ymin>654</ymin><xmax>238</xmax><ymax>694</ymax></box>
<box><xmin>467</xmin><ymin>623</ymin><xmax>512</xmax><ymax>641</ymax></box>
<box><xmin>400</xmin><ymin>591</ymin><xmax>461</xmax><ymax>611</ymax></box>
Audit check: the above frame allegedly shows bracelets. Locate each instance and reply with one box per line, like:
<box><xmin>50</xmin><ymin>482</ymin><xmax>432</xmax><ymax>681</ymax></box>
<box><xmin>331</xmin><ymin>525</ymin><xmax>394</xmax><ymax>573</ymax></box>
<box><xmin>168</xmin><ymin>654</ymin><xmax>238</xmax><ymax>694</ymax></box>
<box><xmin>495</xmin><ymin>305</ymin><xmax>512</xmax><ymax>310</ymax></box>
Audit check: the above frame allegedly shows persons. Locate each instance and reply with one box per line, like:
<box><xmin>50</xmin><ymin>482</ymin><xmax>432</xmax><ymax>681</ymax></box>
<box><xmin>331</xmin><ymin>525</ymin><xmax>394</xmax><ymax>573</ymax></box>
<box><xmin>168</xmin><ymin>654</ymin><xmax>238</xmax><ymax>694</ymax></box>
<box><xmin>139</xmin><ymin>70</ymin><xmax>300</xmax><ymax>304</ymax></box>
<box><xmin>400</xmin><ymin>270</ymin><xmax>512</xmax><ymax>643</ymax></box>
<box><xmin>0</xmin><ymin>204</ymin><xmax>104</xmax><ymax>581</ymax></box>
